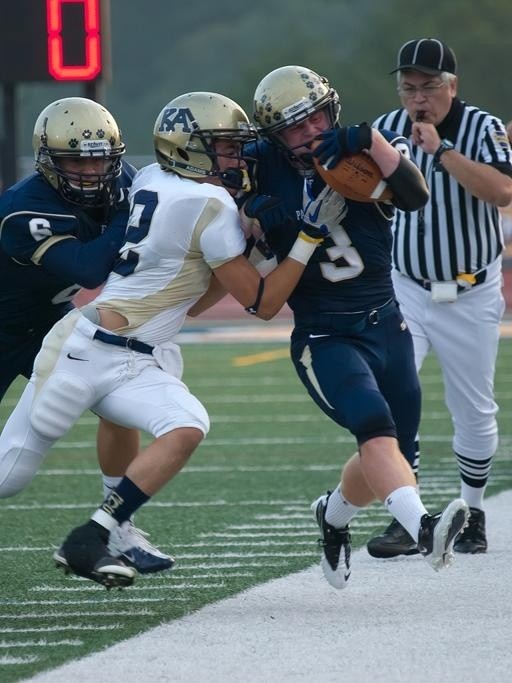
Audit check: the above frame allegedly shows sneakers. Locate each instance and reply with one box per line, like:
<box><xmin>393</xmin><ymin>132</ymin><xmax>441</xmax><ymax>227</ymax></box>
<box><xmin>311</xmin><ymin>489</ymin><xmax>351</xmax><ymax>589</ymax></box>
<box><xmin>367</xmin><ymin>500</ymin><xmax>488</xmax><ymax>571</ymax></box>
<box><xmin>52</xmin><ymin>518</ymin><xmax>175</xmax><ymax>591</ymax></box>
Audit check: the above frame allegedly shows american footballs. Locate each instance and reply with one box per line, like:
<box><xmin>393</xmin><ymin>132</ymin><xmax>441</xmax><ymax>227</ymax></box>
<box><xmin>312</xmin><ymin>139</ymin><xmax>395</xmax><ymax>202</ymax></box>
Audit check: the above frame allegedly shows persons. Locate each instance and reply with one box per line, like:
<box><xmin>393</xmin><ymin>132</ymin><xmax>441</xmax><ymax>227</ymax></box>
<box><xmin>0</xmin><ymin>94</ymin><xmax>177</xmax><ymax>573</ymax></box>
<box><xmin>365</xmin><ymin>35</ymin><xmax>511</xmax><ymax>557</ymax></box>
<box><xmin>0</xmin><ymin>89</ymin><xmax>347</xmax><ymax>592</ymax></box>
<box><xmin>239</xmin><ymin>63</ymin><xmax>471</xmax><ymax>588</ymax></box>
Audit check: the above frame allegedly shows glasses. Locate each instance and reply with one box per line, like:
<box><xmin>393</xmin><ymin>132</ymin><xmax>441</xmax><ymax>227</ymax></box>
<box><xmin>401</xmin><ymin>83</ymin><xmax>445</xmax><ymax>96</ymax></box>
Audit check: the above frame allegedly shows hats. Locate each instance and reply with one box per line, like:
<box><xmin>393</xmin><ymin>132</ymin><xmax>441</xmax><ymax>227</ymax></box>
<box><xmin>389</xmin><ymin>38</ymin><xmax>458</xmax><ymax>76</ymax></box>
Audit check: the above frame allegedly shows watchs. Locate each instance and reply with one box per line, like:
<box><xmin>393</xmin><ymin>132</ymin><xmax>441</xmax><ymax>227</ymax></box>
<box><xmin>429</xmin><ymin>137</ymin><xmax>454</xmax><ymax>164</ymax></box>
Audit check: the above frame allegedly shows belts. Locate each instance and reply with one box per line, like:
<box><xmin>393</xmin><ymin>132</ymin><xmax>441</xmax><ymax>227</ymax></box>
<box><xmin>411</xmin><ymin>269</ymin><xmax>486</xmax><ymax>293</ymax></box>
<box><xmin>93</xmin><ymin>330</ymin><xmax>154</xmax><ymax>354</ymax></box>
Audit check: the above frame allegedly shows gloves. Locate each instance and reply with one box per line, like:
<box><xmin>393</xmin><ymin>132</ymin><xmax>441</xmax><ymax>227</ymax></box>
<box><xmin>298</xmin><ymin>178</ymin><xmax>348</xmax><ymax>244</ymax></box>
<box><xmin>312</xmin><ymin>121</ymin><xmax>371</xmax><ymax>170</ymax></box>
<box><xmin>243</xmin><ymin>193</ymin><xmax>300</xmax><ymax>253</ymax></box>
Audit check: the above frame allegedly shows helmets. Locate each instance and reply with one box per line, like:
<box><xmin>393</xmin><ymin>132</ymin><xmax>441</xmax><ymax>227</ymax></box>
<box><xmin>153</xmin><ymin>91</ymin><xmax>263</xmax><ymax>195</ymax></box>
<box><xmin>253</xmin><ymin>64</ymin><xmax>341</xmax><ymax>179</ymax></box>
<box><xmin>32</xmin><ymin>97</ymin><xmax>126</xmax><ymax>211</ymax></box>
